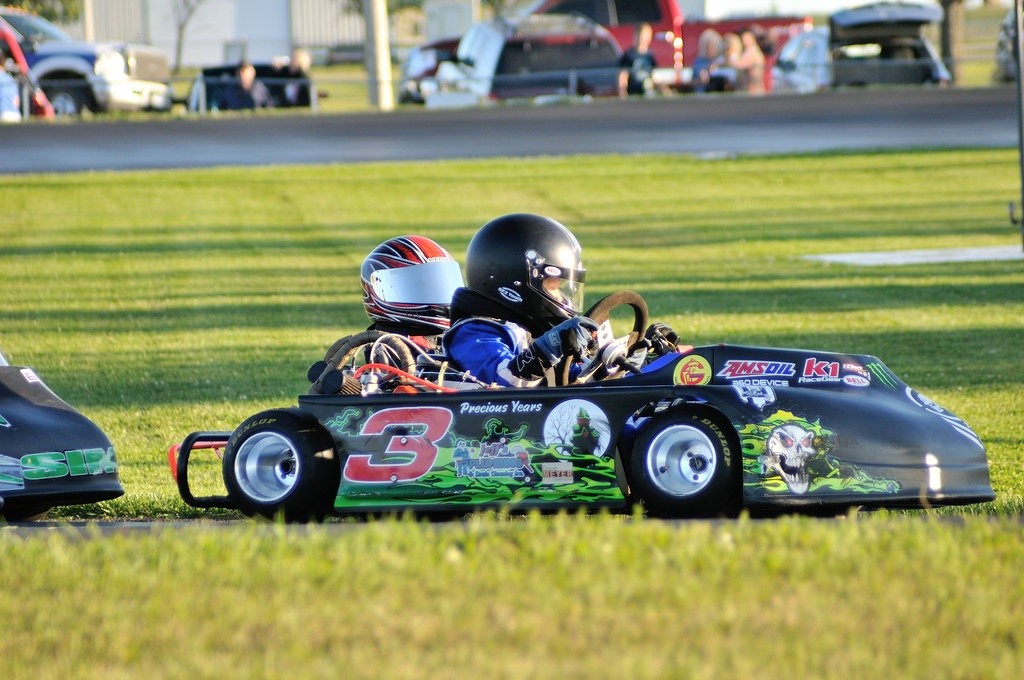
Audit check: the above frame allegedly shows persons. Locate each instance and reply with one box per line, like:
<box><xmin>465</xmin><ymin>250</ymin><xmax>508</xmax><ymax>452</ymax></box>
<box><xmin>361</xmin><ymin>233</ymin><xmax>467</xmax><ymax>393</ymax></box>
<box><xmin>442</xmin><ymin>213</ymin><xmax>646</xmax><ymax>393</ymax></box>
<box><xmin>0</xmin><ymin>22</ymin><xmax>769</xmax><ymax>125</ymax></box>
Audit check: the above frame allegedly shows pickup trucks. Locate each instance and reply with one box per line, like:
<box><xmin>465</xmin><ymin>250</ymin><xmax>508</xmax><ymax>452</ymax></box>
<box><xmin>399</xmin><ymin>0</ymin><xmax>813</xmax><ymax>94</ymax></box>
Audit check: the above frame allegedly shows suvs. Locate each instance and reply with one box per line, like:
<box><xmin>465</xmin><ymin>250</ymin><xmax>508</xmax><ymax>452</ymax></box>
<box><xmin>1</xmin><ymin>8</ymin><xmax>174</xmax><ymax>115</ymax></box>
<box><xmin>401</xmin><ymin>30</ymin><xmax>624</xmax><ymax>106</ymax></box>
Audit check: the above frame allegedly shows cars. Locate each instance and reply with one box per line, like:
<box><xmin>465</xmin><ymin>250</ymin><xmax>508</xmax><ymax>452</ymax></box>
<box><xmin>826</xmin><ymin>1</ymin><xmax>951</xmax><ymax>88</ymax></box>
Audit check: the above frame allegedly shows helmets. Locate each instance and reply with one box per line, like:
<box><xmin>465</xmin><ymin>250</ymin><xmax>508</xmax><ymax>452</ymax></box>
<box><xmin>465</xmin><ymin>213</ymin><xmax>585</xmax><ymax>330</ymax></box>
<box><xmin>360</xmin><ymin>236</ymin><xmax>465</xmax><ymax>337</ymax></box>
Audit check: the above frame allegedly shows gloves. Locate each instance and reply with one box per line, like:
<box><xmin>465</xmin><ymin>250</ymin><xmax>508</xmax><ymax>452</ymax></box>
<box><xmin>624</xmin><ymin>346</ymin><xmax>648</xmax><ymax>371</ymax></box>
<box><xmin>532</xmin><ymin>314</ymin><xmax>599</xmax><ymax>367</ymax></box>
